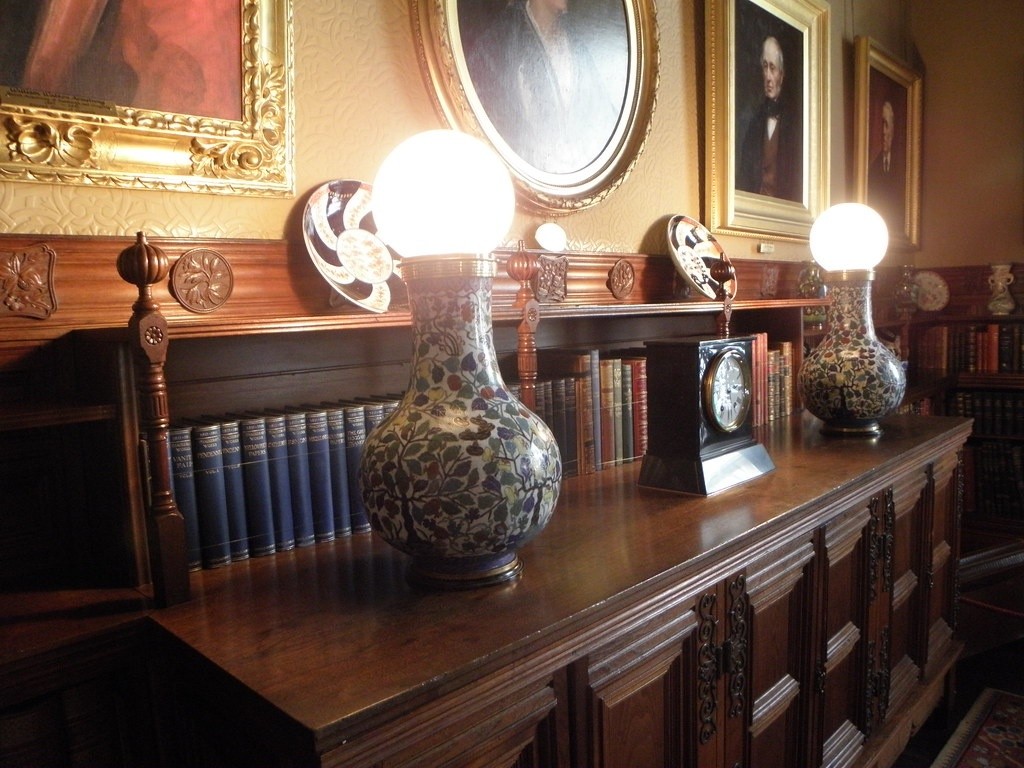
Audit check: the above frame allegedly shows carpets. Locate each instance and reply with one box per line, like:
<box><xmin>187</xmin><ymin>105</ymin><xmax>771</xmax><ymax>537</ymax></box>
<box><xmin>927</xmin><ymin>685</ymin><xmax>1024</xmax><ymax>768</ymax></box>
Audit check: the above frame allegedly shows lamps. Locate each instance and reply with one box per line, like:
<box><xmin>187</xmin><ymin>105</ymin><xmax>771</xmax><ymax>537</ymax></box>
<box><xmin>347</xmin><ymin>129</ymin><xmax>560</xmax><ymax>587</ymax></box>
<box><xmin>794</xmin><ymin>202</ymin><xmax>906</xmax><ymax>439</ymax></box>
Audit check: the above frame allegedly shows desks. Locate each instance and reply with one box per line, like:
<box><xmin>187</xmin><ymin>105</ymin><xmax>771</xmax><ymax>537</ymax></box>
<box><xmin>142</xmin><ymin>415</ymin><xmax>977</xmax><ymax>768</ymax></box>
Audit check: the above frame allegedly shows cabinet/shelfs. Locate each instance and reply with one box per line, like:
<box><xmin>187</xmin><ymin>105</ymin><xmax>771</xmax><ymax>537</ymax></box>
<box><xmin>0</xmin><ymin>318</ymin><xmax>1023</xmax><ymax>768</ymax></box>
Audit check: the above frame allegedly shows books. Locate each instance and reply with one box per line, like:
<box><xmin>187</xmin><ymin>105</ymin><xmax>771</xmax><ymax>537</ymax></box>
<box><xmin>502</xmin><ymin>349</ymin><xmax>648</xmax><ymax>480</ymax></box>
<box><xmin>163</xmin><ymin>390</ymin><xmax>410</xmax><ymax>573</ymax></box>
<box><xmin>745</xmin><ymin>334</ymin><xmax>796</xmax><ymax>428</ymax></box>
<box><xmin>890</xmin><ymin>322</ymin><xmax>1024</xmax><ymax>522</ymax></box>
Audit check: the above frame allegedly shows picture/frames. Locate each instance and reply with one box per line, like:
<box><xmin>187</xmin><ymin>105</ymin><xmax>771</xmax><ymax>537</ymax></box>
<box><xmin>849</xmin><ymin>38</ymin><xmax>923</xmax><ymax>249</ymax></box>
<box><xmin>703</xmin><ymin>0</ymin><xmax>830</xmax><ymax>242</ymax></box>
<box><xmin>0</xmin><ymin>0</ymin><xmax>298</xmax><ymax>200</ymax></box>
<box><xmin>411</xmin><ymin>1</ymin><xmax>662</xmax><ymax>218</ymax></box>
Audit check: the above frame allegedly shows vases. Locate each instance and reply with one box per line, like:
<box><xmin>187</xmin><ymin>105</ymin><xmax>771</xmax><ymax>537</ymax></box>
<box><xmin>987</xmin><ymin>263</ymin><xmax>1015</xmax><ymax>316</ymax></box>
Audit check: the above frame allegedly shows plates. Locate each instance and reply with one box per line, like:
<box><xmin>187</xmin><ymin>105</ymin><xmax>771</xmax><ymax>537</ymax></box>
<box><xmin>302</xmin><ymin>179</ymin><xmax>410</xmax><ymax>313</ymax></box>
<box><xmin>911</xmin><ymin>271</ymin><xmax>949</xmax><ymax>311</ymax></box>
<box><xmin>667</xmin><ymin>214</ymin><xmax>737</xmax><ymax>301</ymax></box>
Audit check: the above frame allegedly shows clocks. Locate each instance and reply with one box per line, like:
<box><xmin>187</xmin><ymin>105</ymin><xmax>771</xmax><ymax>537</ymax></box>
<box><xmin>702</xmin><ymin>343</ymin><xmax>754</xmax><ymax>433</ymax></box>
